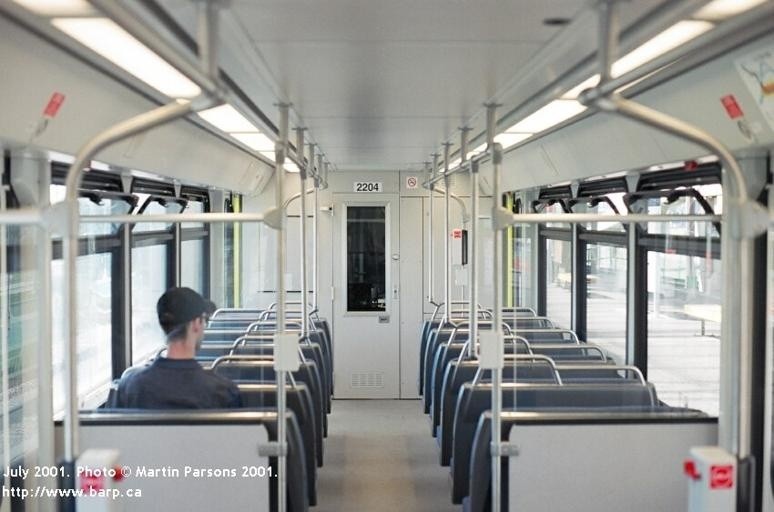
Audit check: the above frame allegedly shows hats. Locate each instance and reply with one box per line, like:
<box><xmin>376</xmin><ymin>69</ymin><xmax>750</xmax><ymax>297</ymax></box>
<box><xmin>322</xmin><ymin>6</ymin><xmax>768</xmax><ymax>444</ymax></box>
<box><xmin>157</xmin><ymin>287</ymin><xmax>216</xmax><ymax>326</ymax></box>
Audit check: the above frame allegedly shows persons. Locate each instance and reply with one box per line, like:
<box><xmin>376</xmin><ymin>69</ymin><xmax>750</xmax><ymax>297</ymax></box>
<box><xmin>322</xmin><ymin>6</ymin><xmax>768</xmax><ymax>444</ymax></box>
<box><xmin>113</xmin><ymin>287</ymin><xmax>243</xmax><ymax>411</ymax></box>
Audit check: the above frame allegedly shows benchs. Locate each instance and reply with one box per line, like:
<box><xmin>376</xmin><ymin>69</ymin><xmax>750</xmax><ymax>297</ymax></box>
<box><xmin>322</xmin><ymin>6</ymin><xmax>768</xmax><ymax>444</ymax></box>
<box><xmin>51</xmin><ymin>297</ymin><xmax>335</xmax><ymax>509</ymax></box>
<box><xmin>418</xmin><ymin>299</ymin><xmax>718</xmax><ymax>512</ymax></box>
